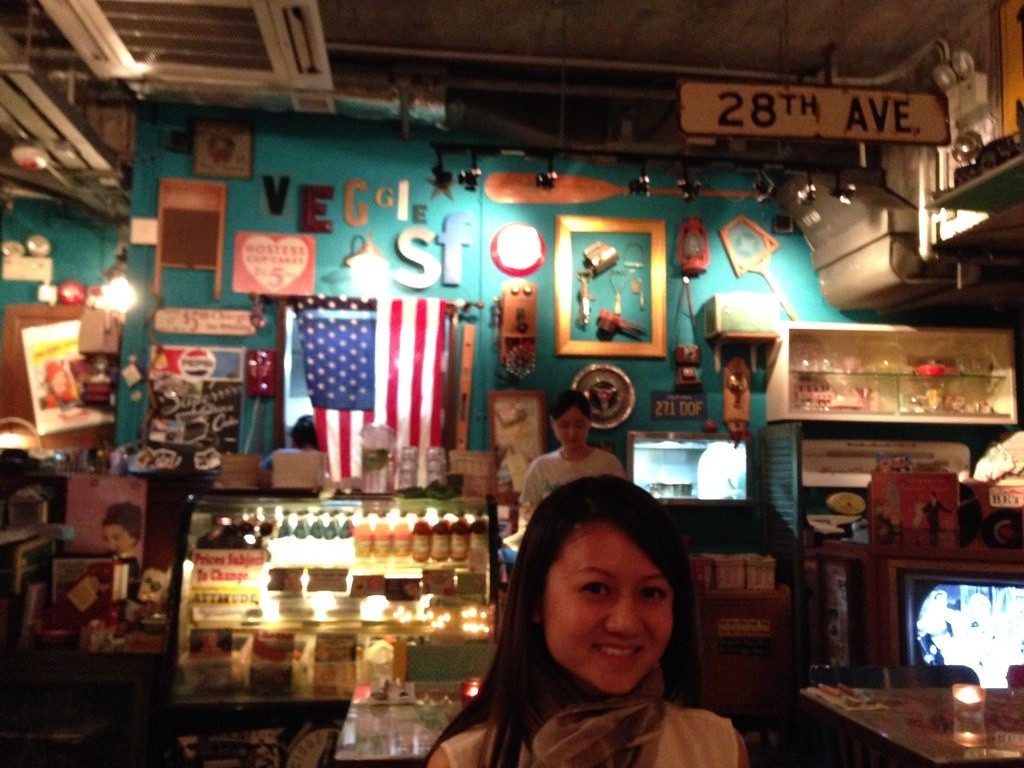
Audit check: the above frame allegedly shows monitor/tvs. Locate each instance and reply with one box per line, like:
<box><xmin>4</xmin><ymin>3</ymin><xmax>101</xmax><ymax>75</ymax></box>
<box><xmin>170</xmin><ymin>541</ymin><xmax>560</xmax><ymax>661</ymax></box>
<box><xmin>903</xmin><ymin>571</ymin><xmax>1024</xmax><ymax>688</ymax></box>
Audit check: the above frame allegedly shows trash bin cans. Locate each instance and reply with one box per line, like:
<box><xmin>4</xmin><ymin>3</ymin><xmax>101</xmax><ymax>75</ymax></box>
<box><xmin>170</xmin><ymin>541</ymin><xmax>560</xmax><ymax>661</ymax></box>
<box><xmin>694</xmin><ymin>582</ymin><xmax>790</xmax><ymax>736</ymax></box>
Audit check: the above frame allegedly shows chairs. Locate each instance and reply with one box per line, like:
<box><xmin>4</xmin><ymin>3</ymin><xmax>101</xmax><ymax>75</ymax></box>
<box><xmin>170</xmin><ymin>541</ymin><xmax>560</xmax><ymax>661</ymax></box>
<box><xmin>809</xmin><ymin>665</ymin><xmax>980</xmax><ymax>768</ymax></box>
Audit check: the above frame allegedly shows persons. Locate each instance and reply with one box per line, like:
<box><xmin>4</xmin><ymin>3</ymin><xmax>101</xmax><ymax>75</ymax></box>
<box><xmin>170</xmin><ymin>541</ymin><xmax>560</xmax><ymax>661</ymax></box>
<box><xmin>262</xmin><ymin>415</ymin><xmax>326</xmax><ymax>486</ymax></box>
<box><xmin>100</xmin><ymin>502</ymin><xmax>143</xmax><ymax>552</ymax></box>
<box><xmin>917</xmin><ymin>590</ymin><xmax>1024</xmax><ymax>690</ymax></box>
<box><xmin>519</xmin><ymin>390</ymin><xmax>627</xmax><ymax>508</ymax></box>
<box><xmin>425</xmin><ymin>477</ymin><xmax>750</xmax><ymax>768</ymax></box>
<box><xmin>922</xmin><ymin>492</ymin><xmax>953</xmax><ymax>527</ymax></box>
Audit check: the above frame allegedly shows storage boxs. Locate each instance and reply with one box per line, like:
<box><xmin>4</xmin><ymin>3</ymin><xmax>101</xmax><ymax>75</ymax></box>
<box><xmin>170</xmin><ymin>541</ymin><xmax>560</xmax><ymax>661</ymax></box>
<box><xmin>961</xmin><ymin>479</ymin><xmax>1024</xmax><ymax>551</ymax></box>
<box><xmin>871</xmin><ymin>471</ymin><xmax>960</xmax><ymax>548</ymax></box>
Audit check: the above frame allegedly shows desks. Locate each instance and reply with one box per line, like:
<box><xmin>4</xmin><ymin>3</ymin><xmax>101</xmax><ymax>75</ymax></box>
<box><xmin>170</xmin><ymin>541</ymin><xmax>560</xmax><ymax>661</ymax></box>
<box><xmin>797</xmin><ymin>686</ymin><xmax>1024</xmax><ymax>768</ymax></box>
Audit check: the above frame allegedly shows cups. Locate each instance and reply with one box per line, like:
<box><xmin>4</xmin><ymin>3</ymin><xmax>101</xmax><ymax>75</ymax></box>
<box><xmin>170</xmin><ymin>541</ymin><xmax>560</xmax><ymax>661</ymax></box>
<box><xmin>362</xmin><ymin>450</ymin><xmax>394</xmax><ymax>494</ymax></box>
<box><xmin>952</xmin><ymin>686</ymin><xmax>986</xmax><ymax>719</ymax></box>
<box><xmin>954</xmin><ymin>715</ymin><xmax>985</xmax><ymax>746</ymax></box>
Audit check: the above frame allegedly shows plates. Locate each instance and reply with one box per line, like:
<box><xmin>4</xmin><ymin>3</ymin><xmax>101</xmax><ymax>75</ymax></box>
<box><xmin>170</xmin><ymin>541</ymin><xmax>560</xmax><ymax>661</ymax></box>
<box><xmin>827</xmin><ymin>492</ymin><xmax>865</xmax><ymax>514</ymax></box>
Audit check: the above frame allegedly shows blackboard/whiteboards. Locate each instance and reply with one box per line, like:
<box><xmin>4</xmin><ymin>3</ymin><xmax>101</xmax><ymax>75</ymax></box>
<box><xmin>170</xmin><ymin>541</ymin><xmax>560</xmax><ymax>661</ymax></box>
<box><xmin>154</xmin><ymin>178</ymin><xmax>227</xmax><ymax>269</ymax></box>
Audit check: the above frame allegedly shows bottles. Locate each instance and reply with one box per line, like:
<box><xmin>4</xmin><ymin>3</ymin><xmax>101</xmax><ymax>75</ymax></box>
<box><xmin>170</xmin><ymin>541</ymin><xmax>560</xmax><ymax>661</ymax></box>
<box><xmin>197</xmin><ymin>513</ymin><xmax>486</xmax><ymax>562</ymax></box>
<box><xmin>426</xmin><ymin>448</ymin><xmax>448</xmax><ymax>486</ymax></box>
<box><xmin>398</xmin><ymin>446</ymin><xmax>419</xmax><ymax>489</ymax></box>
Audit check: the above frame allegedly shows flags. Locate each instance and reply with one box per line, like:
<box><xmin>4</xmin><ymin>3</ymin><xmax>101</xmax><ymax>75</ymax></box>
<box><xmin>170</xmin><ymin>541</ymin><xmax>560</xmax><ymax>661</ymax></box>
<box><xmin>291</xmin><ymin>295</ymin><xmax>448</xmax><ymax>483</ymax></box>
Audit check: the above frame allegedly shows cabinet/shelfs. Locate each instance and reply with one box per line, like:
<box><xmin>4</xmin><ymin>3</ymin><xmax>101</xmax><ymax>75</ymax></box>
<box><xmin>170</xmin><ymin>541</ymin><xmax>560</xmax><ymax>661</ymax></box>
<box><xmin>698</xmin><ymin>585</ymin><xmax>793</xmax><ymax>746</ymax></box>
<box><xmin>765</xmin><ymin>319</ymin><xmax>1019</xmax><ymax>425</ymax></box>
<box><xmin>148</xmin><ymin>488</ymin><xmax>499</xmax><ymax>768</ymax></box>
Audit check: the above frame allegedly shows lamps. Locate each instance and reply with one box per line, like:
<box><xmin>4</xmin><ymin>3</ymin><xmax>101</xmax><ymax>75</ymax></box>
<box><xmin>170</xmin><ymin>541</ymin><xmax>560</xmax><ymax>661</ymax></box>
<box><xmin>536</xmin><ymin>158</ymin><xmax>557</xmax><ymax>189</ymax></box>
<box><xmin>676</xmin><ymin>164</ymin><xmax>702</xmax><ymax>203</ymax></box>
<box><xmin>430</xmin><ymin>152</ymin><xmax>453</xmax><ymax>189</ymax></box>
<box><xmin>457</xmin><ymin>155</ymin><xmax>481</xmax><ymax>193</ymax></box>
<box><xmin>2</xmin><ymin>235</ymin><xmax>53</xmax><ymax>283</ymax></box>
<box><xmin>750</xmin><ymin>164</ymin><xmax>775</xmax><ymax>202</ymax></box>
<box><xmin>934</xmin><ymin>51</ymin><xmax>989</xmax><ymax>123</ymax></box>
<box><xmin>830</xmin><ymin>165</ymin><xmax>857</xmax><ymax>207</ymax></box>
<box><xmin>795</xmin><ymin>170</ymin><xmax>818</xmax><ymax>208</ymax></box>
<box><xmin>628</xmin><ymin>160</ymin><xmax>650</xmax><ymax>196</ymax></box>
